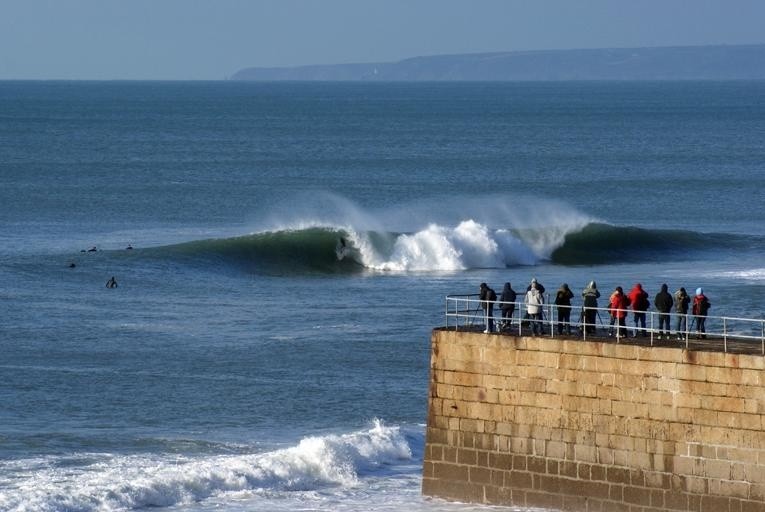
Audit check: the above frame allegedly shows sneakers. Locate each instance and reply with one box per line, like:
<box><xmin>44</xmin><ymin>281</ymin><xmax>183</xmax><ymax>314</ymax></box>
<box><xmin>623</xmin><ymin>334</ymin><xmax>625</xmax><ymax>338</ymax></box>
<box><xmin>696</xmin><ymin>337</ymin><xmax>699</xmax><ymax>339</ymax></box>
<box><xmin>682</xmin><ymin>337</ymin><xmax>685</xmax><ymax>341</ymax></box>
<box><xmin>484</xmin><ymin>330</ymin><xmax>492</xmax><ymax>333</ymax></box>
<box><xmin>616</xmin><ymin>335</ymin><xmax>622</xmax><ymax>338</ymax></box>
<box><xmin>678</xmin><ymin>337</ymin><xmax>681</xmax><ymax>340</ymax></box>
<box><xmin>631</xmin><ymin>335</ymin><xmax>636</xmax><ymax>338</ymax></box>
<box><xmin>667</xmin><ymin>336</ymin><xmax>670</xmax><ymax>339</ymax></box>
<box><xmin>658</xmin><ymin>336</ymin><xmax>662</xmax><ymax>339</ymax></box>
<box><xmin>609</xmin><ymin>332</ymin><xmax>612</xmax><ymax>336</ymax></box>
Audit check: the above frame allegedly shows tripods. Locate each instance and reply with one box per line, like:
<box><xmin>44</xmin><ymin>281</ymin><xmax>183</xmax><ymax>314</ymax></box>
<box><xmin>470</xmin><ymin>303</ymin><xmax>497</xmax><ymax>327</ymax></box>
<box><xmin>576</xmin><ymin>309</ymin><xmax>605</xmax><ymax>331</ymax></box>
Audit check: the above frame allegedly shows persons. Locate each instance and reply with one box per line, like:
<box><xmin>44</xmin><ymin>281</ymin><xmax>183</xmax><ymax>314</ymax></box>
<box><xmin>478</xmin><ymin>282</ymin><xmax>497</xmax><ymax>334</ymax></box>
<box><xmin>556</xmin><ymin>283</ymin><xmax>574</xmax><ymax>335</ymax></box>
<box><xmin>673</xmin><ymin>287</ymin><xmax>690</xmax><ymax>341</ymax></box>
<box><xmin>524</xmin><ymin>283</ymin><xmax>544</xmax><ymax>337</ymax></box>
<box><xmin>581</xmin><ymin>281</ymin><xmax>600</xmax><ymax>333</ymax></box>
<box><xmin>608</xmin><ymin>286</ymin><xmax>622</xmax><ymax>336</ymax></box>
<box><xmin>339</xmin><ymin>235</ymin><xmax>347</xmax><ymax>253</ymax></box>
<box><xmin>611</xmin><ymin>287</ymin><xmax>630</xmax><ymax>339</ymax></box>
<box><xmin>522</xmin><ymin>278</ymin><xmax>546</xmax><ymax>327</ymax></box>
<box><xmin>106</xmin><ymin>275</ymin><xmax>118</xmax><ymax>289</ymax></box>
<box><xmin>499</xmin><ymin>282</ymin><xmax>516</xmax><ymax>330</ymax></box>
<box><xmin>628</xmin><ymin>284</ymin><xmax>649</xmax><ymax>338</ymax></box>
<box><xmin>654</xmin><ymin>283</ymin><xmax>673</xmax><ymax>340</ymax></box>
<box><xmin>692</xmin><ymin>287</ymin><xmax>708</xmax><ymax>340</ymax></box>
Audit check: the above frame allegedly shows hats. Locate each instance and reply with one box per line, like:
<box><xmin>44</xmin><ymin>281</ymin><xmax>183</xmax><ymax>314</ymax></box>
<box><xmin>531</xmin><ymin>278</ymin><xmax>537</xmax><ymax>282</ymax></box>
<box><xmin>590</xmin><ymin>280</ymin><xmax>596</xmax><ymax>288</ymax></box>
